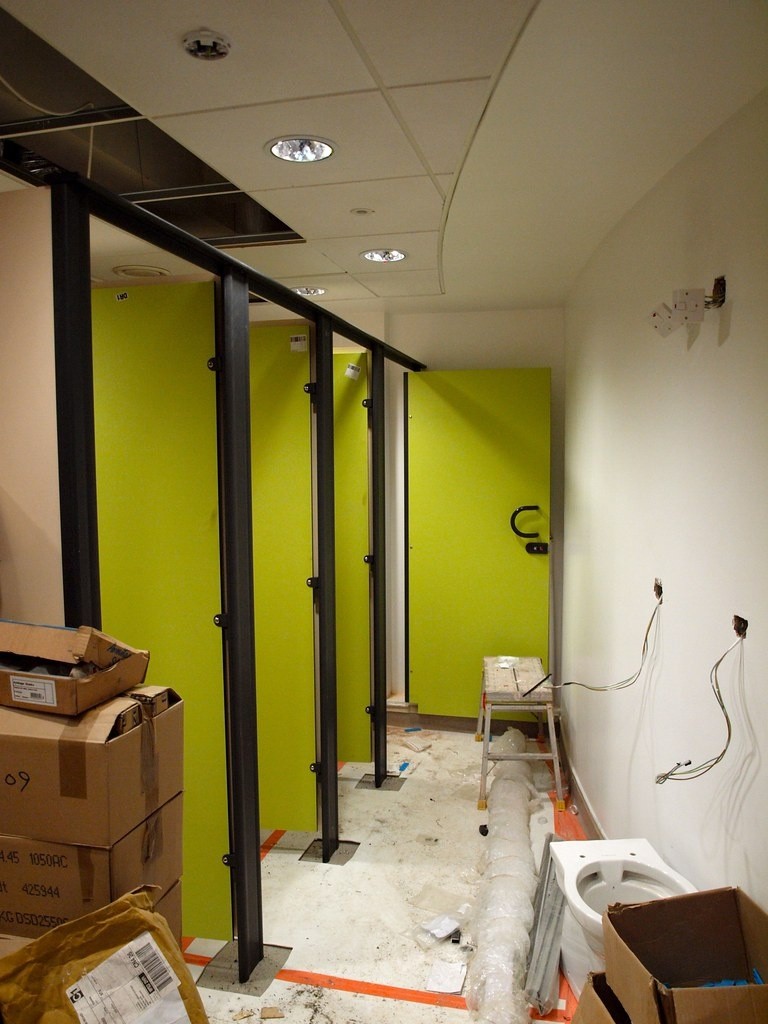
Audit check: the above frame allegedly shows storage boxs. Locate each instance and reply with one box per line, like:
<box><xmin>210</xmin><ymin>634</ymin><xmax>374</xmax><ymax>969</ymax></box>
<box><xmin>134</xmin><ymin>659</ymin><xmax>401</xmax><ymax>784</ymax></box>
<box><xmin>571</xmin><ymin>887</ymin><xmax>768</xmax><ymax>1024</ymax></box>
<box><xmin>1</xmin><ymin>619</ymin><xmax>184</xmax><ymax>955</ymax></box>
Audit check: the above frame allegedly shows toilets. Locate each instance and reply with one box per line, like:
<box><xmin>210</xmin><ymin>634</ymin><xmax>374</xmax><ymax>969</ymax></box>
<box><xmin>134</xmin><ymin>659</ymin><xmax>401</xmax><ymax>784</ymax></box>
<box><xmin>547</xmin><ymin>837</ymin><xmax>700</xmax><ymax>1004</ymax></box>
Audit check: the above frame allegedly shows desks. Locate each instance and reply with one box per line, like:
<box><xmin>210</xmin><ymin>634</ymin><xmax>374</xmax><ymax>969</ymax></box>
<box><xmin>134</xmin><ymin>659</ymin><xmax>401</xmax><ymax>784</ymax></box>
<box><xmin>475</xmin><ymin>656</ymin><xmax>566</xmax><ymax>813</ymax></box>
